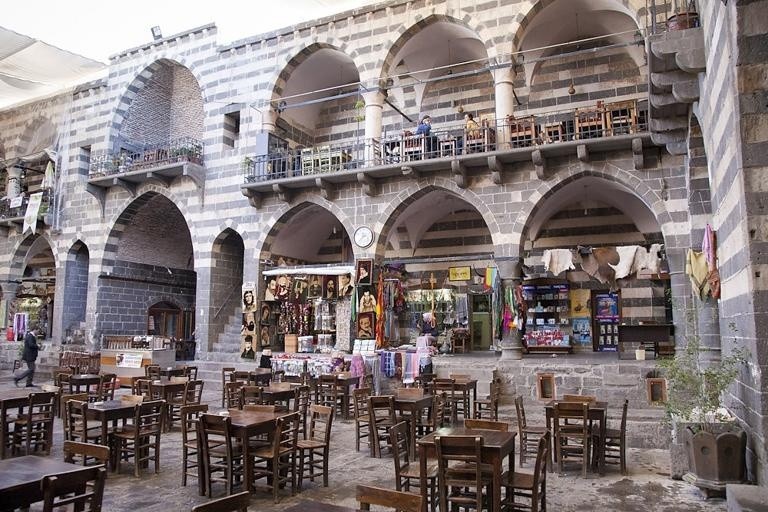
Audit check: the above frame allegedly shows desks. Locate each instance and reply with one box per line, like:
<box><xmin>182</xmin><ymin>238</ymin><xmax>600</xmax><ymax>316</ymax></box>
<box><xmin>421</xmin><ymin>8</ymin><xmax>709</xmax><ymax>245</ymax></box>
<box><xmin>617</xmin><ymin>325</ymin><xmax>675</xmax><ymax>359</ymax></box>
<box><xmin>544</xmin><ymin>400</ymin><xmax>608</xmax><ymax>475</ymax></box>
<box><xmin>282</xmin><ymin>500</ymin><xmax>353</xmax><ymax>511</ymax></box>
<box><xmin>1</xmin><ymin>454</ymin><xmax>88</xmax><ymax>512</ymax></box>
<box><xmin>416</xmin><ymin>427</ymin><xmax>517</xmax><ymax>511</ymax></box>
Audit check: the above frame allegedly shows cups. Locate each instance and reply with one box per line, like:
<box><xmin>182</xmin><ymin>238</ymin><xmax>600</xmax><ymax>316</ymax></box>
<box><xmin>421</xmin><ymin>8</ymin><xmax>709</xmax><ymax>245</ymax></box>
<box><xmin>166</xmin><ymin>366</ymin><xmax>172</xmax><ymax>371</ymax></box>
<box><xmin>638</xmin><ymin>321</ymin><xmax>643</xmax><ymax>325</ymax></box>
<box><xmin>621</xmin><ymin>322</ymin><xmax>626</xmax><ymax>325</ymax></box>
<box><xmin>219</xmin><ymin>411</ymin><xmax>230</xmax><ymax>420</ymax></box>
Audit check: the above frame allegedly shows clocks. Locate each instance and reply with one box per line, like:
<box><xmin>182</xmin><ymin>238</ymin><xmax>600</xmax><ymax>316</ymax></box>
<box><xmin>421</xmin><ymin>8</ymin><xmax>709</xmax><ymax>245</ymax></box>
<box><xmin>353</xmin><ymin>225</ymin><xmax>375</xmax><ymax>250</ymax></box>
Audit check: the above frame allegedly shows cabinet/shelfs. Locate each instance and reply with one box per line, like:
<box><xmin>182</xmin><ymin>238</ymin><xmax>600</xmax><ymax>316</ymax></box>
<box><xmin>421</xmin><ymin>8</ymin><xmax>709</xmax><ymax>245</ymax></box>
<box><xmin>473</xmin><ymin>312</ymin><xmax>492</xmax><ymax>351</ymax></box>
<box><xmin>522</xmin><ymin>277</ymin><xmax>573</xmax><ymax>353</ymax></box>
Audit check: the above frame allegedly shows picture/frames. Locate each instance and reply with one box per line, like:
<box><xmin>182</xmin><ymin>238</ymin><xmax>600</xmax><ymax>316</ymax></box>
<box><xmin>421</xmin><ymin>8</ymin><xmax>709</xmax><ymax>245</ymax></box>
<box><xmin>355</xmin><ymin>311</ymin><xmax>376</xmax><ymax>339</ymax></box>
<box><xmin>354</xmin><ymin>259</ymin><xmax>373</xmax><ymax>286</ymax></box>
<box><xmin>448</xmin><ymin>266</ymin><xmax>471</xmax><ymax>281</ymax></box>
<box><xmin>240</xmin><ymin>274</ymin><xmax>354</xmax><ymax>361</ymax></box>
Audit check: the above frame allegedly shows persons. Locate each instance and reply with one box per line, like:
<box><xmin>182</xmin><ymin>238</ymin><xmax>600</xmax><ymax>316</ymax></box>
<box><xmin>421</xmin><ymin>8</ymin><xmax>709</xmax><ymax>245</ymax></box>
<box><xmin>415</xmin><ymin>330</ymin><xmax>438</xmax><ymax>390</ymax></box>
<box><xmin>241</xmin><ymin>264</ymin><xmax>375</xmax><ymax>369</ymax></box>
<box><xmin>452</xmin><ymin>113</ymin><xmax>482</xmax><ymax>157</ymax></box>
<box><xmin>412</xmin><ymin>113</ymin><xmax>442</xmax><ymax>157</ymax></box>
<box><xmin>13</xmin><ymin>324</ymin><xmax>43</xmax><ymax>388</ymax></box>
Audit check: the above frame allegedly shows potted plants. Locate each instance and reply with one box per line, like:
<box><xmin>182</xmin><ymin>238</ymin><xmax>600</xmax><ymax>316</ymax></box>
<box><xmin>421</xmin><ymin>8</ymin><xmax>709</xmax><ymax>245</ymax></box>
<box><xmin>655</xmin><ymin>290</ymin><xmax>752</xmax><ymax>480</ymax></box>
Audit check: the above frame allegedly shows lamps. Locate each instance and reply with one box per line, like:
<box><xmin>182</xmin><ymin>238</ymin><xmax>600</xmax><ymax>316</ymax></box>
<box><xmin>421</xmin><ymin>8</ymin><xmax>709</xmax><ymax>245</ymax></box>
<box><xmin>12</xmin><ymin>163</ymin><xmax>45</xmax><ymax>179</ymax></box>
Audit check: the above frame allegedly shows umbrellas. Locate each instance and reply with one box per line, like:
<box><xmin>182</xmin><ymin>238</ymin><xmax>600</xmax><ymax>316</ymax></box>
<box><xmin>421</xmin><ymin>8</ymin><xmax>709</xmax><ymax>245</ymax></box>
<box><xmin>40</xmin><ymin>160</ymin><xmax>57</xmax><ymax>213</ymax></box>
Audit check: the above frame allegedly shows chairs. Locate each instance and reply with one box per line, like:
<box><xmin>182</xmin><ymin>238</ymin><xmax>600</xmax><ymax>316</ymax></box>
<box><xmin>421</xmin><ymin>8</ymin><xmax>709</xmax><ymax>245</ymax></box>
<box><xmin>591</xmin><ymin>399</ymin><xmax>628</xmax><ymax>475</ymax></box>
<box><xmin>640</xmin><ymin>321</ymin><xmax>659</xmax><ymax>359</ymax></box>
<box><xmin>191</xmin><ymin>491</ymin><xmax>249</xmax><ymax>511</ymax></box>
<box><xmin>554</xmin><ymin>402</ymin><xmax>591</xmax><ymax>479</ymax></box>
<box><xmin>0</xmin><ymin>351</ymin><xmax>208</xmax><ymax>477</ymax></box>
<box><xmin>354</xmin><ymin>484</ymin><xmax>422</xmax><ymax>511</ymax></box>
<box><xmin>181</xmin><ymin>335</ymin><xmax>359</xmax><ymax>503</ymax></box>
<box><xmin>472</xmin><ymin>378</ymin><xmax>499</xmax><ymax>422</ymax></box>
<box><xmin>561</xmin><ymin>395</ymin><xmax>596</xmax><ymax>468</ymax></box>
<box><xmin>450</xmin><ymin>419</ymin><xmax>508</xmax><ymax>474</ymax></box>
<box><xmin>500</xmin><ymin>427</ymin><xmax>551</xmax><ymax>511</ymax></box>
<box><xmin>300</xmin><ymin>98</ymin><xmax>647</xmax><ymax>175</ymax></box>
<box><xmin>515</xmin><ymin>396</ymin><xmax>552</xmax><ymax>468</ymax></box>
<box><xmin>60</xmin><ymin>440</ymin><xmax>110</xmax><ymax>503</ymax></box>
<box><xmin>389</xmin><ymin>421</ymin><xmax>439</xmax><ymax>512</ymax></box>
<box><xmin>41</xmin><ymin>464</ymin><xmax>106</xmax><ymax>511</ymax></box>
<box><xmin>341</xmin><ymin>366</ymin><xmax>477</xmax><ymax>461</ymax></box>
<box><xmin>433</xmin><ymin>434</ymin><xmax>493</xmax><ymax>512</ymax></box>
